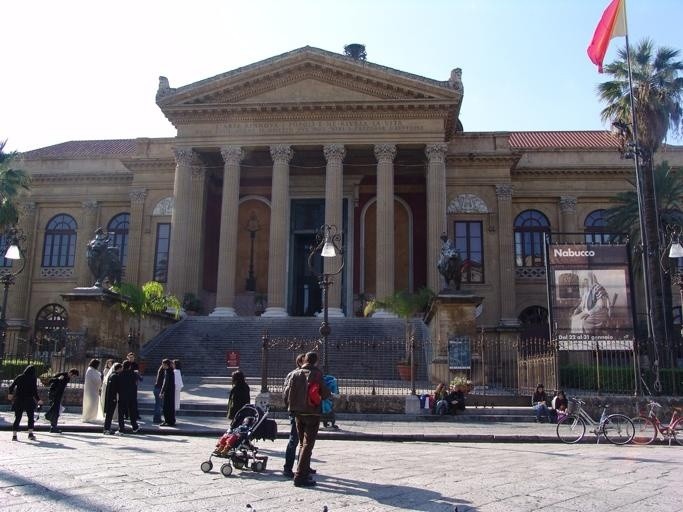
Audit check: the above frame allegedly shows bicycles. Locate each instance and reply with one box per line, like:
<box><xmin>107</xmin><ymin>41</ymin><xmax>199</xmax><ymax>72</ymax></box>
<box><xmin>555</xmin><ymin>397</ymin><xmax>634</xmax><ymax>445</ymax></box>
<box><xmin>627</xmin><ymin>400</ymin><xmax>682</xmax><ymax>445</ymax></box>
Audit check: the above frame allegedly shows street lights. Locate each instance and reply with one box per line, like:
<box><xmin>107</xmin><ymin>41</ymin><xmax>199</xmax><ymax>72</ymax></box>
<box><xmin>660</xmin><ymin>223</ymin><xmax>682</xmax><ymax>376</ymax></box>
<box><xmin>306</xmin><ymin>222</ymin><xmax>346</xmax><ymax>375</ymax></box>
<box><xmin>611</xmin><ymin>116</ymin><xmax>654</xmax><ymax>365</ymax></box>
<box><xmin>0</xmin><ymin>225</ymin><xmax>29</xmax><ymax>368</ymax></box>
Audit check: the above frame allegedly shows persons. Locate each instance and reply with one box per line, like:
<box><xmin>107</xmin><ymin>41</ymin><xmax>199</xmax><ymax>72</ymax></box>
<box><xmin>433</xmin><ymin>383</ymin><xmax>448</xmax><ymax>415</ymax></box>
<box><xmin>8</xmin><ymin>365</ymin><xmax>42</xmax><ymax>440</ymax></box>
<box><xmin>556</xmin><ymin>404</ymin><xmax>566</xmax><ymax>424</ymax></box>
<box><xmin>551</xmin><ymin>390</ymin><xmax>568</xmax><ymax>423</ymax></box>
<box><xmin>154</xmin><ymin>359</ymin><xmax>184</xmax><ymax>427</ymax></box>
<box><xmin>227</xmin><ymin>370</ymin><xmax>249</xmax><ymax>419</ymax></box>
<box><xmin>285</xmin><ymin>353</ymin><xmax>330</xmax><ymax>487</ymax></box>
<box><xmin>570</xmin><ymin>272</ymin><xmax>613</xmax><ymax>336</ymax></box>
<box><xmin>449</xmin><ymin>385</ymin><xmax>465</xmax><ymax>416</ymax></box>
<box><xmin>45</xmin><ymin>368</ymin><xmax>79</xmax><ymax>432</ymax></box>
<box><xmin>532</xmin><ymin>384</ymin><xmax>552</xmax><ymax>423</ymax></box>
<box><xmin>84</xmin><ymin>352</ymin><xmax>142</xmax><ymax>434</ymax></box>
<box><xmin>282</xmin><ymin>354</ymin><xmax>316</xmax><ymax>477</ymax></box>
<box><xmin>214</xmin><ymin>416</ymin><xmax>254</xmax><ymax>455</ymax></box>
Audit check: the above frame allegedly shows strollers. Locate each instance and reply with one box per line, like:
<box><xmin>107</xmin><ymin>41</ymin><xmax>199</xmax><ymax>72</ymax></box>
<box><xmin>200</xmin><ymin>402</ymin><xmax>279</xmax><ymax>477</ymax></box>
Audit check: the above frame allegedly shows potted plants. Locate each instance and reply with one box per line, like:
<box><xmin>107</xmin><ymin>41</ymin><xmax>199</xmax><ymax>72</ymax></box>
<box><xmin>449</xmin><ymin>376</ymin><xmax>473</xmax><ymax>394</ymax></box>
<box><xmin>379</xmin><ymin>281</ymin><xmax>434</xmax><ymax>380</ymax></box>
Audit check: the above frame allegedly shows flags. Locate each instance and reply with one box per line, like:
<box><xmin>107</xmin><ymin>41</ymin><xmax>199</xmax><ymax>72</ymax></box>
<box><xmin>587</xmin><ymin>1</ymin><xmax>627</xmax><ymax>73</ymax></box>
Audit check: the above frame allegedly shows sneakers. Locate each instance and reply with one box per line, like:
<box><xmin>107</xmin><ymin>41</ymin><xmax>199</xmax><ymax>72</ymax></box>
<box><xmin>294</xmin><ymin>468</ymin><xmax>316</xmax><ymax>486</ymax></box>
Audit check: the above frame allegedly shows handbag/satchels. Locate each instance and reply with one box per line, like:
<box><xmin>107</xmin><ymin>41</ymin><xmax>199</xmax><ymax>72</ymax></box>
<box><xmin>308</xmin><ymin>384</ymin><xmax>321</xmax><ymax>406</ymax></box>
